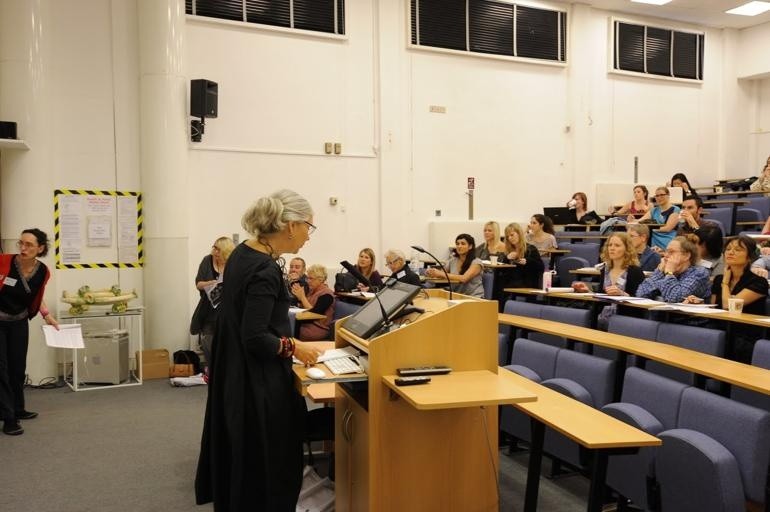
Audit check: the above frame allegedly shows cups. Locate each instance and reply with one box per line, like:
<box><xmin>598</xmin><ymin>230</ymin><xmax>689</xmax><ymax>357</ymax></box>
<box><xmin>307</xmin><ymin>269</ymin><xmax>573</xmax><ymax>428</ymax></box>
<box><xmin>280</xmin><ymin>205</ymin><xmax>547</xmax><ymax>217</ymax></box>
<box><xmin>679</xmin><ymin>210</ymin><xmax>686</xmax><ymax>223</ymax></box>
<box><xmin>568</xmin><ymin>199</ymin><xmax>576</xmax><ymax>208</ymax></box>
<box><xmin>542</xmin><ymin>272</ymin><xmax>552</xmax><ymax>290</ymax></box>
<box><xmin>715</xmin><ymin>186</ymin><xmax>723</xmax><ymax>193</ymax></box>
<box><xmin>727</xmin><ymin>298</ymin><xmax>744</xmax><ymax>314</ymax></box>
<box><xmin>490</xmin><ymin>256</ymin><xmax>498</xmax><ymax>266</ymax></box>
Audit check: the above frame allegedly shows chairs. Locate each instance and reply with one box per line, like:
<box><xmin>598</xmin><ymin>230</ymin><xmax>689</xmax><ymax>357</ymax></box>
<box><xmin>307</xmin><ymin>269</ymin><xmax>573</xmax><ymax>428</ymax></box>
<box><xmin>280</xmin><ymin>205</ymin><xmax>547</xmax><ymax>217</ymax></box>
<box><xmin>277</xmin><ymin>183</ymin><xmax>770</xmax><ymax>512</ymax></box>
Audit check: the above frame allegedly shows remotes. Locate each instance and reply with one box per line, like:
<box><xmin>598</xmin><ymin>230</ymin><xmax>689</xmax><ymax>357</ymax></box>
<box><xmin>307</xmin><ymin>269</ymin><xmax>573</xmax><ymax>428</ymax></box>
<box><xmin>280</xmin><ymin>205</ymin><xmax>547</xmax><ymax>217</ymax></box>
<box><xmin>394</xmin><ymin>375</ymin><xmax>431</xmax><ymax>387</ymax></box>
<box><xmin>396</xmin><ymin>366</ymin><xmax>453</xmax><ymax>376</ymax></box>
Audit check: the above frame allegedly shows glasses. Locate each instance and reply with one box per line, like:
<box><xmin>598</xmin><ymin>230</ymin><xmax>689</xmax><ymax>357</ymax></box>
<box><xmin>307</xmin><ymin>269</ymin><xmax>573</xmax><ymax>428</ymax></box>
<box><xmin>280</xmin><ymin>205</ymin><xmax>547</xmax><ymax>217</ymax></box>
<box><xmin>305</xmin><ymin>220</ymin><xmax>317</xmax><ymax>235</ymax></box>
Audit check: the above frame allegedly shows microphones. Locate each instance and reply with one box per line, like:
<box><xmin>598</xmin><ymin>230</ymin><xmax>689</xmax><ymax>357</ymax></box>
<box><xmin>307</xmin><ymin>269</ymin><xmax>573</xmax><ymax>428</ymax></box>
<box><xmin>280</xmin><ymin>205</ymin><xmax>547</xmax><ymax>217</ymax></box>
<box><xmin>340</xmin><ymin>260</ymin><xmax>400</xmax><ymax>342</ymax></box>
<box><xmin>409</xmin><ymin>245</ymin><xmax>453</xmax><ymax>301</ymax></box>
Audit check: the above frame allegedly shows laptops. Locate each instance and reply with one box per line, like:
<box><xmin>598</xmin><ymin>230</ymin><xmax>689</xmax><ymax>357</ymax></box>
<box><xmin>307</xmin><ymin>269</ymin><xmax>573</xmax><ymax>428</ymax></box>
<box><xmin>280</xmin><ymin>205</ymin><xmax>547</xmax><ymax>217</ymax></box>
<box><xmin>667</xmin><ymin>187</ymin><xmax>683</xmax><ymax>204</ymax></box>
<box><xmin>544</xmin><ymin>207</ymin><xmax>568</xmax><ymax>224</ymax></box>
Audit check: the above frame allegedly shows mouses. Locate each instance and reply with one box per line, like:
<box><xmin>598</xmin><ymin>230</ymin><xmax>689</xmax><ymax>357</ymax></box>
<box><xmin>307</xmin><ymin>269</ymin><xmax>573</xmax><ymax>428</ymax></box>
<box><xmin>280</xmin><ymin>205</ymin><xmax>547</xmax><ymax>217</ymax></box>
<box><xmin>306</xmin><ymin>367</ymin><xmax>326</xmax><ymax>380</ymax></box>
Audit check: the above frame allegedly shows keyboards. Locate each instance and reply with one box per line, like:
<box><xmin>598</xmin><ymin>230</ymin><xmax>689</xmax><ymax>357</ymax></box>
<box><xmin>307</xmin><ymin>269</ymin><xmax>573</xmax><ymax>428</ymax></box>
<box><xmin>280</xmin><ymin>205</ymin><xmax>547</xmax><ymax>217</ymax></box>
<box><xmin>325</xmin><ymin>355</ymin><xmax>365</xmax><ymax>376</ymax></box>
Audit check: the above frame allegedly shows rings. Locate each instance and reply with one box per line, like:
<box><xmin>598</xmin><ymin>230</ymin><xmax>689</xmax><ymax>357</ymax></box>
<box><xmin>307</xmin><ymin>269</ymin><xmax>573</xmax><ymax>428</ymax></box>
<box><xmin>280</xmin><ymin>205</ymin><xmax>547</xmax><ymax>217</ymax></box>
<box><xmin>308</xmin><ymin>360</ymin><xmax>315</xmax><ymax>365</ymax></box>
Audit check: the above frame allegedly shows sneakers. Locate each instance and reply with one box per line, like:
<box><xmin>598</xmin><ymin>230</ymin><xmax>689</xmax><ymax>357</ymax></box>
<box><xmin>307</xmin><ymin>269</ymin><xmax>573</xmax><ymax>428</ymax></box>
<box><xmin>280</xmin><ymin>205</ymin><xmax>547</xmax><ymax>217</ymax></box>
<box><xmin>3</xmin><ymin>421</ymin><xmax>25</xmax><ymax>435</ymax></box>
<box><xmin>17</xmin><ymin>410</ymin><xmax>38</xmax><ymax>420</ymax></box>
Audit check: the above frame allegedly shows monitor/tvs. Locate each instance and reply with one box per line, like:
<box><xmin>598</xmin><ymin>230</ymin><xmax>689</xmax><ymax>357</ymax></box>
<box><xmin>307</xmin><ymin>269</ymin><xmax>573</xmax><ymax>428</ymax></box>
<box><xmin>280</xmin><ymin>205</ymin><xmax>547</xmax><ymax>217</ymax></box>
<box><xmin>340</xmin><ymin>279</ymin><xmax>421</xmax><ymax>341</ymax></box>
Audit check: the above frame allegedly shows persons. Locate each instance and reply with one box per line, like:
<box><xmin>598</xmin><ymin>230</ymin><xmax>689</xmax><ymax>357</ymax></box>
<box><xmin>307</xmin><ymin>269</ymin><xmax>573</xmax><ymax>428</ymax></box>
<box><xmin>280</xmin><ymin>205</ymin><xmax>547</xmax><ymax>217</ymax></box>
<box><xmin>361</xmin><ymin>250</ymin><xmax>422</xmax><ymax>293</ymax></box>
<box><xmin>193</xmin><ymin>189</ymin><xmax>318</xmax><ymax>512</ymax></box>
<box><xmin>290</xmin><ymin>266</ymin><xmax>335</xmax><ymax>341</ymax></box>
<box><xmin>284</xmin><ymin>257</ymin><xmax>309</xmax><ymax>304</ymax></box>
<box><xmin>425</xmin><ymin>154</ymin><xmax>770</xmax><ymax>317</ymax></box>
<box><xmin>0</xmin><ymin>229</ymin><xmax>61</xmax><ymax>435</ymax></box>
<box><xmin>188</xmin><ymin>236</ymin><xmax>239</xmax><ymax>385</ymax></box>
<box><xmin>342</xmin><ymin>248</ymin><xmax>383</xmax><ymax>289</ymax></box>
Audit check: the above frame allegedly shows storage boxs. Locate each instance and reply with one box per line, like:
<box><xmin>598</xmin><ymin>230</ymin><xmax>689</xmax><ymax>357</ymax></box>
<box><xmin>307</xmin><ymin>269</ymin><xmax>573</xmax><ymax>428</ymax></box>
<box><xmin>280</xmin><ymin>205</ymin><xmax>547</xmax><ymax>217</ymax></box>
<box><xmin>76</xmin><ymin>327</ymin><xmax>130</xmax><ymax>385</ymax></box>
<box><xmin>136</xmin><ymin>347</ymin><xmax>170</xmax><ymax>382</ymax></box>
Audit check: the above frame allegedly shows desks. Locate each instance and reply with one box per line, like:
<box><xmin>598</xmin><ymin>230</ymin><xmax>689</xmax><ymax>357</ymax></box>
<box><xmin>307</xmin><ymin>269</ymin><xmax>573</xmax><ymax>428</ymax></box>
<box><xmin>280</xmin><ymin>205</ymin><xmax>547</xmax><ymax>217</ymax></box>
<box><xmin>55</xmin><ymin>308</ymin><xmax>145</xmax><ymax>393</ymax></box>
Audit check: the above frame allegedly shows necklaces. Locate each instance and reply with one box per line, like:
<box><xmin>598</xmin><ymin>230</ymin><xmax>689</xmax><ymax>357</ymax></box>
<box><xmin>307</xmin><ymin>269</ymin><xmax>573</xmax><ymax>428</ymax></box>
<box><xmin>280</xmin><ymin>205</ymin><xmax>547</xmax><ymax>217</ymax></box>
<box><xmin>259</xmin><ymin>235</ymin><xmax>291</xmax><ymax>291</ymax></box>
<box><xmin>17</xmin><ymin>258</ymin><xmax>38</xmax><ymax>277</ymax></box>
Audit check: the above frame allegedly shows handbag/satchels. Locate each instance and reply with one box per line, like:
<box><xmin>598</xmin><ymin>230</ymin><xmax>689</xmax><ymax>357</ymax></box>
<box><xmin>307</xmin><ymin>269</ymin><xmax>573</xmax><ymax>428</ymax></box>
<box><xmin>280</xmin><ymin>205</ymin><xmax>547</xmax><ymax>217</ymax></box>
<box><xmin>168</xmin><ymin>352</ymin><xmax>194</xmax><ymax>378</ymax></box>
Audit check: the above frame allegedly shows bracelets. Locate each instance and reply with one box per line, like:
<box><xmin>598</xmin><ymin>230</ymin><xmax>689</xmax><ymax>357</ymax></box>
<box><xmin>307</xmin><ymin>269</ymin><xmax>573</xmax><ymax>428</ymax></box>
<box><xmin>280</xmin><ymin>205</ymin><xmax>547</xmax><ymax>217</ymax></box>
<box><xmin>279</xmin><ymin>336</ymin><xmax>295</xmax><ymax>358</ymax></box>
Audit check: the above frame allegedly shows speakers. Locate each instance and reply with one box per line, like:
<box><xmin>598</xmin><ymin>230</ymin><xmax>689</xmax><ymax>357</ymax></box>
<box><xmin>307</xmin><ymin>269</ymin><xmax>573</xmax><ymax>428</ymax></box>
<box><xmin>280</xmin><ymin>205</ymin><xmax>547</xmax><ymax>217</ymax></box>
<box><xmin>0</xmin><ymin>121</ymin><xmax>17</xmax><ymax>139</ymax></box>
<box><xmin>191</xmin><ymin>79</ymin><xmax>218</xmax><ymax>118</ymax></box>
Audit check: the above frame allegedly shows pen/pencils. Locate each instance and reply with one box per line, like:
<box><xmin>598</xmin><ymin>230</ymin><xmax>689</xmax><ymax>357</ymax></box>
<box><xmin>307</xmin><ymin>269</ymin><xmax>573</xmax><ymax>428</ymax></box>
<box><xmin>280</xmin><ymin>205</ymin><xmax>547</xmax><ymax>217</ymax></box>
<box><xmin>349</xmin><ymin>357</ymin><xmax>360</xmax><ymax>365</ymax></box>
<box><xmin>683</xmin><ymin>297</ymin><xmax>705</xmax><ymax>301</ymax></box>
